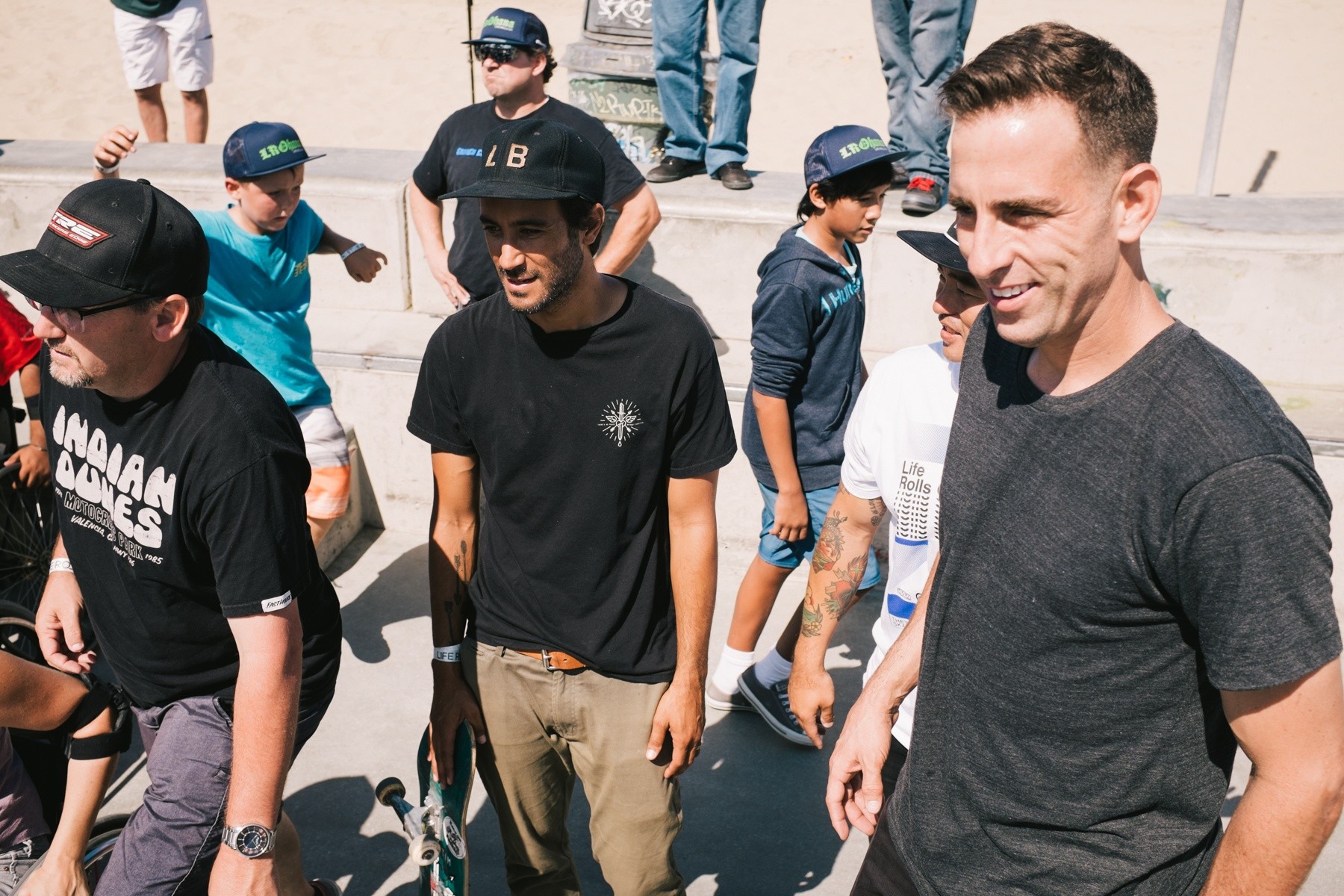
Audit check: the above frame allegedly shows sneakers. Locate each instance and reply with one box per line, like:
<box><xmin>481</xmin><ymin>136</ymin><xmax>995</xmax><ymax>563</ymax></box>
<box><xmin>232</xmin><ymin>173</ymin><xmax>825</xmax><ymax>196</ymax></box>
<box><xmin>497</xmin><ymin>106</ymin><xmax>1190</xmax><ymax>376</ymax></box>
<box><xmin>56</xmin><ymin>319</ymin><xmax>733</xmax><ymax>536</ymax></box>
<box><xmin>704</xmin><ymin>678</ymin><xmax>755</xmax><ymax>711</ymax></box>
<box><xmin>737</xmin><ymin>661</ymin><xmax>822</xmax><ymax>748</ymax></box>
<box><xmin>900</xmin><ymin>177</ymin><xmax>944</xmax><ymax>212</ymax></box>
<box><xmin>888</xmin><ymin>162</ymin><xmax>909</xmax><ymax>189</ymax></box>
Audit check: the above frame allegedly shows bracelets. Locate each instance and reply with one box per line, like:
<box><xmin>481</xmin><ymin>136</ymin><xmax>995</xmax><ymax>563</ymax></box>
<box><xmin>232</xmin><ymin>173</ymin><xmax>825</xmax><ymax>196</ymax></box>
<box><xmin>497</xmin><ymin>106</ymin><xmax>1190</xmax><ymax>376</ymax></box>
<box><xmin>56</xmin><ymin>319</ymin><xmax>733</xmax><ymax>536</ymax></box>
<box><xmin>434</xmin><ymin>644</ymin><xmax>461</xmax><ymax>662</ymax></box>
<box><xmin>31</xmin><ymin>443</ymin><xmax>48</xmax><ymax>452</ymax></box>
<box><xmin>49</xmin><ymin>558</ymin><xmax>75</xmax><ymax>574</ymax></box>
<box><xmin>93</xmin><ymin>157</ymin><xmax>121</xmax><ymax>174</ymax></box>
<box><xmin>341</xmin><ymin>243</ymin><xmax>366</xmax><ymax>260</ymax></box>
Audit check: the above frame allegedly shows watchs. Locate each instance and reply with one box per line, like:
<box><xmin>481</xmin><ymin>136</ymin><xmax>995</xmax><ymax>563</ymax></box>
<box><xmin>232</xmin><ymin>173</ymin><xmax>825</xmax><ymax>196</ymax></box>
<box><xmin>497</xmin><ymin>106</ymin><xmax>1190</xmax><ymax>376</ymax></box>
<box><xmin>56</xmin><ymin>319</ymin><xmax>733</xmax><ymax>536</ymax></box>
<box><xmin>221</xmin><ymin>823</ymin><xmax>278</xmax><ymax>858</ymax></box>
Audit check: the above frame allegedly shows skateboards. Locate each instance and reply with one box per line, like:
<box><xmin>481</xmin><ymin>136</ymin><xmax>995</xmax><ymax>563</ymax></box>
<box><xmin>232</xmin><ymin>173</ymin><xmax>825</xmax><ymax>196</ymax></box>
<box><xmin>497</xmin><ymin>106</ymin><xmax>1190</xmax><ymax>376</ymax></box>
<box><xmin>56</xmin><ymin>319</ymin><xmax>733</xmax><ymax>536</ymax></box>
<box><xmin>375</xmin><ymin>719</ymin><xmax>476</xmax><ymax>896</ymax></box>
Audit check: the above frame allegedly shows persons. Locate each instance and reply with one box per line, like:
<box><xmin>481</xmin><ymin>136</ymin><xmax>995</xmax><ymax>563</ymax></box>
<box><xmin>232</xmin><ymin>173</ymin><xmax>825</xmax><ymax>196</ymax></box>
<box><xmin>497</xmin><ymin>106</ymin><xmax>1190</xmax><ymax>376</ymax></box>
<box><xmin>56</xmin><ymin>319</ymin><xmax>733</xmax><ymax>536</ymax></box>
<box><xmin>646</xmin><ymin>0</ymin><xmax>766</xmax><ymax>190</ymax></box>
<box><xmin>0</xmin><ymin>290</ymin><xmax>133</xmax><ymax>896</ymax></box>
<box><xmin>406</xmin><ymin>118</ymin><xmax>738</xmax><ymax>896</ymax></box>
<box><xmin>0</xmin><ymin>178</ymin><xmax>342</xmax><ymax>896</ymax></box>
<box><xmin>93</xmin><ymin>119</ymin><xmax>387</xmax><ymax>551</ymax></box>
<box><xmin>787</xmin><ymin>221</ymin><xmax>987</xmax><ymax>896</ymax></box>
<box><xmin>872</xmin><ymin>0</ymin><xmax>977</xmax><ymax>213</ymax></box>
<box><xmin>110</xmin><ymin>0</ymin><xmax>215</xmax><ymax>143</ymax></box>
<box><xmin>405</xmin><ymin>8</ymin><xmax>661</xmax><ymax>311</ymax></box>
<box><xmin>704</xmin><ymin>123</ymin><xmax>909</xmax><ymax>749</ymax></box>
<box><xmin>825</xmin><ymin>22</ymin><xmax>1344</xmax><ymax>896</ymax></box>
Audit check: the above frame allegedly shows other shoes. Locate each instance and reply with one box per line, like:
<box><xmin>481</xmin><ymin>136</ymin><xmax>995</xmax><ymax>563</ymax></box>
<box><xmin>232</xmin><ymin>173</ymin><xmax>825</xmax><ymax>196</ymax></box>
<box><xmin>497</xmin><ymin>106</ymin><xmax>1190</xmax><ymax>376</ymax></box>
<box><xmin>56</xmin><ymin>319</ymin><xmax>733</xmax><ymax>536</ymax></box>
<box><xmin>646</xmin><ymin>155</ymin><xmax>705</xmax><ymax>183</ymax></box>
<box><xmin>711</xmin><ymin>162</ymin><xmax>753</xmax><ymax>189</ymax></box>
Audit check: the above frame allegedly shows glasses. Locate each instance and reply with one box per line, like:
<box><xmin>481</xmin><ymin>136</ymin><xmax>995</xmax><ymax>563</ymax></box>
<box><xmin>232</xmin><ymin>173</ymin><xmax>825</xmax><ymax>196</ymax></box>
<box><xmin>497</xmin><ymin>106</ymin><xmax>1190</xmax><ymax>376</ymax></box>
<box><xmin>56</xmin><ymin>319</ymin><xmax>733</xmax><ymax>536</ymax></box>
<box><xmin>473</xmin><ymin>44</ymin><xmax>540</xmax><ymax>62</ymax></box>
<box><xmin>24</xmin><ymin>294</ymin><xmax>165</xmax><ymax>333</ymax></box>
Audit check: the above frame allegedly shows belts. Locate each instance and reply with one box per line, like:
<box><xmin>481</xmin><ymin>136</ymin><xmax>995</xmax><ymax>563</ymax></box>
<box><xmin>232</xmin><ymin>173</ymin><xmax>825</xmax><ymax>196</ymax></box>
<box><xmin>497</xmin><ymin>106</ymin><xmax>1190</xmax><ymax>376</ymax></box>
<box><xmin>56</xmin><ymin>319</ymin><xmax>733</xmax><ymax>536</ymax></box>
<box><xmin>515</xmin><ymin>649</ymin><xmax>586</xmax><ymax>671</ymax></box>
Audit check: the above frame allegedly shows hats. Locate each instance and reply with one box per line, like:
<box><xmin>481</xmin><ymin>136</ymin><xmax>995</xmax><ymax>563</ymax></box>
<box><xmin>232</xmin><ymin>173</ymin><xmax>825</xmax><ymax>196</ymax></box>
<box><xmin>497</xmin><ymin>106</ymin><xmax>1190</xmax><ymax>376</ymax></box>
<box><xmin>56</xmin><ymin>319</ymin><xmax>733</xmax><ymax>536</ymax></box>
<box><xmin>438</xmin><ymin>119</ymin><xmax>605</xmax><ymax>203</ymax></box>
<box><xmin>804</xmin><ymin>125</ymin><xmax>910</xmax><ymax>186</ymax></box>
<box><xmin>0</xmin><ymin>177</ymin><xmax>209</xmax><ymax>307</ymax></box>
<box><xmin>223</xmin><ymin>121</ymin><xmax>327</xmax><ymax>178</ymax></box>
<box><xmin>461</xmin><ymin>8</ymin><xmax>550</xmax><ymax>53</ymax></box>
<box><xmin>896</xmin><ymin>218</ymin><xmax>971</xmax><ymax>272</ymax></box>
